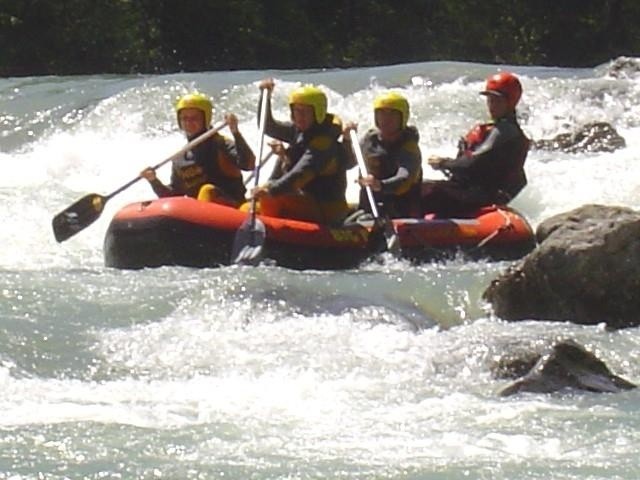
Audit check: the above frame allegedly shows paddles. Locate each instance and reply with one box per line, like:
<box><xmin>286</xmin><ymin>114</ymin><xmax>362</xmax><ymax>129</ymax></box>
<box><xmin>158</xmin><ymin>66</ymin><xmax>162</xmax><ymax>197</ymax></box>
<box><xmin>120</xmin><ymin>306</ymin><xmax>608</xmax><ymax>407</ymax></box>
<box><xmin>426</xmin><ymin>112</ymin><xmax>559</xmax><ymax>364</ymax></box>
<box><xmin>349</xmin><ymin>128</ymin><xmax>402</xmax><ymax>265</ymax></box>
<box><xmin>229</xmin><ymin>88</ymin><xmax>269</xmax><ymax>265</ymax></box>
<box><xmin>52</xmin><ymin>120</ymin><xmax>228</xmax><ymax>244</ymax></box>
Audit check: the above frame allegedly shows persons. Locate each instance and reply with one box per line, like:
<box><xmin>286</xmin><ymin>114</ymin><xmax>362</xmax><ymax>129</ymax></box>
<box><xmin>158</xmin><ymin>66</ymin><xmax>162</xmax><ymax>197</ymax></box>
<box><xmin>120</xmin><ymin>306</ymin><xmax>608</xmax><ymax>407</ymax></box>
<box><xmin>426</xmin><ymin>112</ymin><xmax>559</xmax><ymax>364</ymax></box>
<box><xmin>240</xmin><ymin>78</ymin><xmax>350</xmax><ymax>225</ymax></box>
<box><xmin>423</xmin><ymin>71</ymin><xmax>529</xmax><ymax>219</ymax></box>
<box><xmin>142</xmin><ymin>94</ymin><xmax>255</xmax><ymax>210</ymax></box>
<box><xmin>341</xmin><ymin>94</ymin><xmax>423</xmax><ymax>219</ymax></box>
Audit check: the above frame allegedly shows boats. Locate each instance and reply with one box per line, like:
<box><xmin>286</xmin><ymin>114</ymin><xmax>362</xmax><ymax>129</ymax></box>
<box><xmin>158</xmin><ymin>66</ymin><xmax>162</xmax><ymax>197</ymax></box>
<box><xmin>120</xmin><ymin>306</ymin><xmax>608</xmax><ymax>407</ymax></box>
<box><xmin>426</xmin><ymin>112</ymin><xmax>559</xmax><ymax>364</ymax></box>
<box><xmin>102</xmin><ymin>186</ymin><xmax>537</xmax><ymax>274</ymax></box>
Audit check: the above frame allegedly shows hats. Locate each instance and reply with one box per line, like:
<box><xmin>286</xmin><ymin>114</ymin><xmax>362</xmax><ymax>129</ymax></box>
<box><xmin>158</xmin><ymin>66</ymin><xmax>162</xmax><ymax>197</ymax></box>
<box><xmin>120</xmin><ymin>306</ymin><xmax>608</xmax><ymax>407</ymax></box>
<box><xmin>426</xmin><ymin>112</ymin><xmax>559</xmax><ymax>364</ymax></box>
<box><xmin>479</xmin><ymin>89</ymin><xmax>503</xmax><ymax>97</ymax></box>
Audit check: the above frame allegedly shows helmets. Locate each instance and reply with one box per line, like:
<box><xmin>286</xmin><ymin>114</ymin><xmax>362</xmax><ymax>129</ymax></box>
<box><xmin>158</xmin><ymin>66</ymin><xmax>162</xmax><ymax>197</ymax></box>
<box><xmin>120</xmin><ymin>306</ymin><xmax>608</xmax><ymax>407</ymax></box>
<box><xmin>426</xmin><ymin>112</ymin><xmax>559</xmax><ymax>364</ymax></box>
<box><xmin>289</xmin><ymin>87</ymin><xmax>327</xmax><ymax>125</ymax></box>
<box><xmin>176</xmin><ymin>94</ymin><xmax>212</xmax><ymax>130</ymax></box>
<box><xmin>487</xmin><ymin>72</ymin><xmax>521</xmax><ymax>109</ymax></box>
<box><xmin>374</xmin><ymin>92</ymin><xmax>409</xmax><ymax>130</ymax></box>
